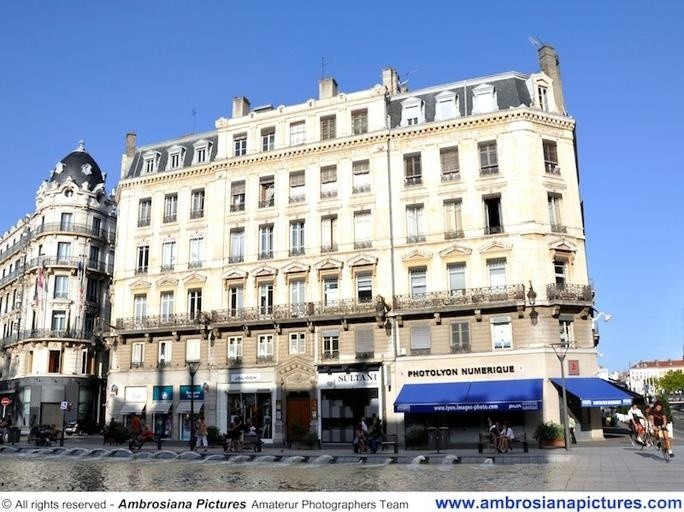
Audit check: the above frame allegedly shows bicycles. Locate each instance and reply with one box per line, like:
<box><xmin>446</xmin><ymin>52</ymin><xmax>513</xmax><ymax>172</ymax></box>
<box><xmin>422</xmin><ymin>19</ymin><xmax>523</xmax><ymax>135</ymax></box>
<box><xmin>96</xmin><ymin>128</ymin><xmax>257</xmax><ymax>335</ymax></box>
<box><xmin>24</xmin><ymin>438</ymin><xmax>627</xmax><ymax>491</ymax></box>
<box><xmin>628</xmin><ymin>419</ymin><xmax>670</xmax><ymax>464</ymax></box>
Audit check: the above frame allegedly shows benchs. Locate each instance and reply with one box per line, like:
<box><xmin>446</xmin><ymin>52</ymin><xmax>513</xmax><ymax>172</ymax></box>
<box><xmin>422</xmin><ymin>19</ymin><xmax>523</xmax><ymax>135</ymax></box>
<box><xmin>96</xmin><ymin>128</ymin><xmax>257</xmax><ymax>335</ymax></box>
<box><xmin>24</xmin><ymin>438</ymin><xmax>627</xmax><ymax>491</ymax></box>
<box><xmin>477</xmin><ymin>430</ymin><xmax>529</xmax><ymax>454</ymax></box>
<box><xmin>241</xmin><ymin>434</ymin><xmax>262</xmax><ymax>452</ymax></box>
<box><xmin>148</xmin><ymin>432</ymin><xmax>161</xmax><ymax>450</ymax></box>
<box><xmin>353</xmin><ymin>432</ymin><xmax>398</xmax><ymax>454</ymax></box>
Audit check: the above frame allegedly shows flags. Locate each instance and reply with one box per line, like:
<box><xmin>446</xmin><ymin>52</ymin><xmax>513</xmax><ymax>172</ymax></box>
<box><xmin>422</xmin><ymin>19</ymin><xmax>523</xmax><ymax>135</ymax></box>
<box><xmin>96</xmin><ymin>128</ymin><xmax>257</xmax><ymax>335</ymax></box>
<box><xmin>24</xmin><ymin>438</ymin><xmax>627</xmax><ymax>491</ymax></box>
<box><xmin>36</xmin><ymin>265</ymin><xmax>44</xmax><ymax>289</ymax></box>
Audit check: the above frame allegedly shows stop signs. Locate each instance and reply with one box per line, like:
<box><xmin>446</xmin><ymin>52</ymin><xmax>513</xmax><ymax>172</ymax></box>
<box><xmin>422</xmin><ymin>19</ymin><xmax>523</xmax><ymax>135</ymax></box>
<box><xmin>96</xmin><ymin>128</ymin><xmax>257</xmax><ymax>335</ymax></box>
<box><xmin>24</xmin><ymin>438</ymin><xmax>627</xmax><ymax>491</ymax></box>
<box><xmin>1</xmin><ymin>398</ymin><xmax>8</xmax><ymax>406</ymax></box>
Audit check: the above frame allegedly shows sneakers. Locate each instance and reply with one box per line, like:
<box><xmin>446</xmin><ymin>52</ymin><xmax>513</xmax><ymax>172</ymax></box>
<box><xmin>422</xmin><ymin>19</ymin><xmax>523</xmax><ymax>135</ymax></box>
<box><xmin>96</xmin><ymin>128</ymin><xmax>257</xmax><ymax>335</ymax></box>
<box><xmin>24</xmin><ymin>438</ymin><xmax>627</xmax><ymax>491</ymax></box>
<box><xmin>636</xmin><ymin>437</ymin><xmax>674</xmax><ymax>458</ymax></box>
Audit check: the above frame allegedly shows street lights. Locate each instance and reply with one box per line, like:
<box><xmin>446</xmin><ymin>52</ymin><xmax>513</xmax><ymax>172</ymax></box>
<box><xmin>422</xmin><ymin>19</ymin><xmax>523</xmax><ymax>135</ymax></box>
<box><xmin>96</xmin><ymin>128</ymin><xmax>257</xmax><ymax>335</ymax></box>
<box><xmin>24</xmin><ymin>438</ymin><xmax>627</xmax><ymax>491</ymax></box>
<box><xmin>185</xmin><ymin>359</ymin><xmax>201</xmax><ymax>450</ymax></box>
<box><xmin>549</xmin><ymin>341</ymin><xmax>576</xmax><ymax>450</ymax></box>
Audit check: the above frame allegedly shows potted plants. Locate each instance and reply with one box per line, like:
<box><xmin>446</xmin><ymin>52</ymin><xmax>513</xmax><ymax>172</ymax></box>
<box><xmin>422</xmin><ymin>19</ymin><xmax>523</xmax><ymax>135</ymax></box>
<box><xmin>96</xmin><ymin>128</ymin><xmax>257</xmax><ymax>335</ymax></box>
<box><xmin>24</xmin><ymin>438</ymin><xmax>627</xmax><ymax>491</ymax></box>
<box><xmin>284</xmin><ymin>416</ymin><xmax>309</xmax><ymax>450</ymax></box>
<box><xmin>204</xmin><ymin>425</ymin><xmax>222</xmax><ymax>447</ymax></box>
<box><xmin>403</xmin><ymin>428</ymin><xmax>430</xmax><ymax>449</ymax></box>
<box><xmin>29</xmin><ymin>421</ymin><xmax>58</xmax><ymax>447</ymax></box>
<box><xmin>534</xmin><ymin>422</ymin><xmax>567</xmax><ymax>448</ymax></box>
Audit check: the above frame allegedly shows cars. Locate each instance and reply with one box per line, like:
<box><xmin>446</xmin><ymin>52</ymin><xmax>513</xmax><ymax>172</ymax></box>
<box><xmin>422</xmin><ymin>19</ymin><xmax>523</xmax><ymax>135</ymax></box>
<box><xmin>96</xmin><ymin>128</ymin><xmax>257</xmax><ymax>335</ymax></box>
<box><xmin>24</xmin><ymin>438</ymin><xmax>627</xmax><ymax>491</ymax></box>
<box><xmin>65</xmin><ymin>419</ymin><xmax>104</xmax><ymax>436</ymax></box>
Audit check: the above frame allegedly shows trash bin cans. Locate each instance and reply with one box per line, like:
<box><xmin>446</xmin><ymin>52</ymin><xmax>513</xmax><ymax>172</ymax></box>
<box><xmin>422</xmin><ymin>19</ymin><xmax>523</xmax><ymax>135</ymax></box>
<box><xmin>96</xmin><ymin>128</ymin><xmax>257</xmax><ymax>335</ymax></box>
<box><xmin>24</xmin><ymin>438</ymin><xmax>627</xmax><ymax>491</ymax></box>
<box><xmin>425</xmin><ymin>427</ymin><xmax>449</xmax><ymax>450</ymax></box>
<box><xmin>8</xmin><ymin>427</ymin><xmax>21</xmax><ymax>442</ymax></box>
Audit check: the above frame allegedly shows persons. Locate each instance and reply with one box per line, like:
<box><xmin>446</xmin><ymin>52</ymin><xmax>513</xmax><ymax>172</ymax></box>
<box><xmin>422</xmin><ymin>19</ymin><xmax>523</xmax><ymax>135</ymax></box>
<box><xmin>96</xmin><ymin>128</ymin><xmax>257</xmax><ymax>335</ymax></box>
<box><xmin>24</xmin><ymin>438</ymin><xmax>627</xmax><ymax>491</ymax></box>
<box><xmin>192</xmin><ymin>415</ymin><xmax>260</xmax><ymax>453</ymax></box>
<box><xmin>627</xmin><ymin>403</ymin><xmax>650</xmax><ymax>448</ymax></box>
<box><xmin>567</xmin><ymin>414</ymin><xmax>576</xmax><ymax>444</ymax></box>
<box><xmin>353</xmin><ymin>413</ymin><xmax>383</xmax><ymax>454</ymax></box>
<box><xmin>648</xmin><ymin>399</ymin><xmax>675</xmax><ymax>459</ymax></box>
<box><xmin>130</xmin><ymin>411</ymin><xmax>142</xmax><ymax>434</ymax></box>
<box><xmin>488</xmin><ymin>422</ymin><xmax>501</xmax><ymax>448</ymax></box>
<box><xmin>130</xmin><ymin>424</ymin><xmax>154</xmax><ymax>450</ymax></box>
<box><xmin>499</xmin><ymin>424</ymin><xmax>514</xmax><ymax>453</ymax></box>
